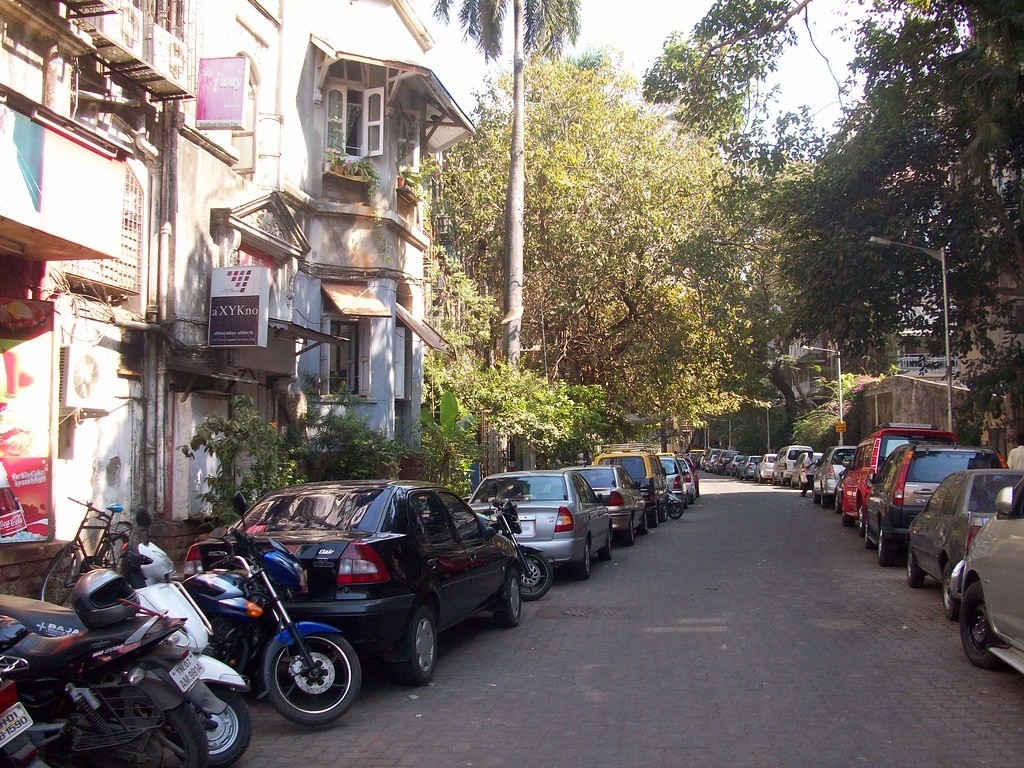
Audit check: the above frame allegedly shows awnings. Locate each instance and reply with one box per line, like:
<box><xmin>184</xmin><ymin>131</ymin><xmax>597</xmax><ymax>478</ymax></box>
<box><xmin>395</xmin><ymin>302</ymin><xmax>457</xmax><ymax>361</ymax></box>
<box><xmin>321</xmin><ymin>283</ymin><xmax>393</xmax><ymax>318</ymax></box>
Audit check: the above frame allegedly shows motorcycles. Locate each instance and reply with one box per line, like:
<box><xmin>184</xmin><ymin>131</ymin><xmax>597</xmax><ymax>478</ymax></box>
<box><xmin>0</xmin><ymin>494</ymin><xmax>361</xmax><ymax>768</ymax></box>
<box><xmin>419</xmin><ymin>481</ymin><xmax>554</xmax><ymax>601</ymax></box>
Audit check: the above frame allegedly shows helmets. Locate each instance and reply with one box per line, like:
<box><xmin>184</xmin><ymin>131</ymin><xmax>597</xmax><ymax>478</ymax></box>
<box><xmin>71</xmin><ymin>569</ymin><xmax>140</xmax><ymax>630</ymax></box>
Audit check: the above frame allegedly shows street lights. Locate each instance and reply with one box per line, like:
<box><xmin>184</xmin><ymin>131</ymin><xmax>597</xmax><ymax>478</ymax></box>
<box><xmin>869</xmin><ymin>236</ymin><xmax>954</xmax><ymax>432</ymax></box>
<box><xmin>801</xmin><ymin>344</ymin><xmax>844</xmax><ymax>447</ymax></box>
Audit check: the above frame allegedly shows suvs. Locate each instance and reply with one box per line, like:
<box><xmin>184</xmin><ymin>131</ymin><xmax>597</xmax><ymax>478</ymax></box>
<box><xmin>790</xmin><ymin>451</ymin><xmax>824</xmax><ymax>490</ymax></box>
<box><xmin>754</xmin><ymin>453</ymin><xmax>778</xmax><ymax>484</ymax></box>
<box><xmin>811</xmin><ymin>445</ymin><xmax>858</xmax><ymax>509</ymax></box>
<box><xmin>841</xmin><ymin>421</ymin><xmax>963</xmax><ymax>538</ymax></box>
<box><xmin>959</xmin><ymin>475</ymin><xmax>1024</xmax><ymax>675</ymax></box>
<box><xmin>865</xmin><ymin>441</ymin><xmax>1010</xmax><ymax>567</ymax></box>
<box><xmin>585</xmin><ymin>449</ymin><xmax>669</xmax><ymax>528</ymax></box>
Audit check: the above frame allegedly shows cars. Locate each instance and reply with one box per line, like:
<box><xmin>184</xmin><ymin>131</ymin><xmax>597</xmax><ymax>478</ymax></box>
<box><xmin>541</xmin><ymin>463</ymin><xmax>648</xmax><ymax>546</ymax></box>
<box><xmin>184</xmin><ymin>480</ymin><xmax>522</xmax><ymax>688</ymax></box>
<box><xmin>650</xmin><ymin>447</ymin><xmax>762</xmax><ymax>507</ymax></box>
<box><xmin>906</xmin><ymin>468</ymin><xmax>1024</xmax><ymax>622</ymax></box>
<box><xmin>461</xmin><ymin>469</ymin><xmax>613</xmax><ymax>581</ymax></box>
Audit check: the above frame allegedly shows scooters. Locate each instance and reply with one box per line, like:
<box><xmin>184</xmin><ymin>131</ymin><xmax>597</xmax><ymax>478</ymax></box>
<box><xmin>666</xmin><ymin>488</ymin><xmax>686</xmax><ymax>520</ymax></box>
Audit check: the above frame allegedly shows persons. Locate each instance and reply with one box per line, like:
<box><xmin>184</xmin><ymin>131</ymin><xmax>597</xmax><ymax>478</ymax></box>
<box><xmin>800</xmin><ymin>452</ymin><xmax>817</xmax><ymax>496</ymax></box>
<box><xmin>1006</xmin><ymin>433</ymin><xmax>1024</xmax><ymax>469</ymax></box>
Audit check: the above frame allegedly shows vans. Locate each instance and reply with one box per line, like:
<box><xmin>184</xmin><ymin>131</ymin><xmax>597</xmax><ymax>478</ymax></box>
<box><xmin>772</xmin><ymin>445</ymin><xmax>814</xmax><ymax>485</ymax></box>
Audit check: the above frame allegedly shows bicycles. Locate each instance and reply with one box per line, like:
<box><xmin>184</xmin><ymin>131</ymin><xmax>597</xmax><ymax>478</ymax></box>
<box><xmin>40</xmin><ymin>497</ymin><xmax>134</xmax><ymax>609</ymax></box>
